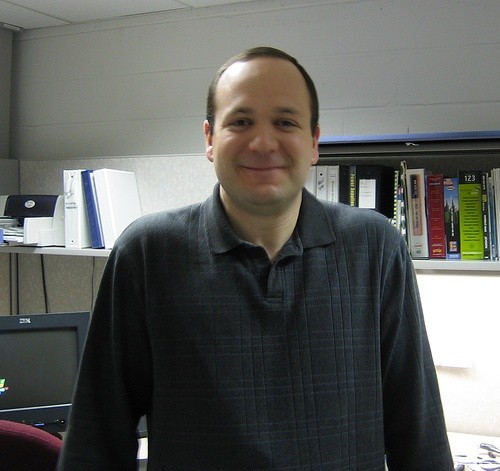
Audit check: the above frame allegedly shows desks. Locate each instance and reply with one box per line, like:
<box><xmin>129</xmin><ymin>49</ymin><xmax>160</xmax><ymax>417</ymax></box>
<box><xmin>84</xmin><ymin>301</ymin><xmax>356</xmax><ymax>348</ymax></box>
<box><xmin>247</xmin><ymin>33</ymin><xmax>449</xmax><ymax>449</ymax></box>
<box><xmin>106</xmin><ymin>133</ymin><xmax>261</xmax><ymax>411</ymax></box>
<box><xmin>0</xmin><ymin>246</ymin><xmax>114</xmax><ymax>316</ymax></box>
<box><xmin>138</xmin><ymin>430</ymin><xmax>500</xmax><ymax>471</ymax></box>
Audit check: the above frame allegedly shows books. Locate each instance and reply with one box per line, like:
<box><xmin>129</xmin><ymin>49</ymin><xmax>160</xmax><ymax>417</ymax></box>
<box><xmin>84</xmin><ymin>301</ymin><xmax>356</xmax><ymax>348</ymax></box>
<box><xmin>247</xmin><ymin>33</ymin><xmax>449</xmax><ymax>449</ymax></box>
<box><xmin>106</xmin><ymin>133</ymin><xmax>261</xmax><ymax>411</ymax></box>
<box><xmin>312</xmin><ymin>164</ymin><xmax>500</xmax><ymax>260</ymax></box>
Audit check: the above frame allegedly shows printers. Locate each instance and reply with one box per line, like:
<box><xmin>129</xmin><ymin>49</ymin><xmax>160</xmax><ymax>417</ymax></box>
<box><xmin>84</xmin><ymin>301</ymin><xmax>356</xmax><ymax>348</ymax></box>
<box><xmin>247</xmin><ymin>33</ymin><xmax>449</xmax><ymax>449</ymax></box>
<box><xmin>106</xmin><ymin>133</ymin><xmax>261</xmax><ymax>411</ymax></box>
<box><xmin>0</xmin><ymin>194</ymin><xmax>66</xmax><ymax>246</ymax></box>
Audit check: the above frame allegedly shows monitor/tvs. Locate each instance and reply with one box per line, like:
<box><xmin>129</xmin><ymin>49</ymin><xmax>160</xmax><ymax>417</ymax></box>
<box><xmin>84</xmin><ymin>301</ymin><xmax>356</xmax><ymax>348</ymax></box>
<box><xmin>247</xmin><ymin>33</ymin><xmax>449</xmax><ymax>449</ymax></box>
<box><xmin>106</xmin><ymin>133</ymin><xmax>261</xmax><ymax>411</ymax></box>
<box><xmin>0</xmin><ymin>311</ymin><xmax>91</xmax><ymax>439</ymax></box>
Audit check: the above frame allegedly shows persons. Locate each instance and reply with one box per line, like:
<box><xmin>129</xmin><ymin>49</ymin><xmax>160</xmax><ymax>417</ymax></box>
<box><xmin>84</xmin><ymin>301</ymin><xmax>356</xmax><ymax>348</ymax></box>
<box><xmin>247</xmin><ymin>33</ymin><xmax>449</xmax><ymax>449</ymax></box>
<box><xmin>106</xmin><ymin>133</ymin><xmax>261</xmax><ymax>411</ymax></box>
<box><xmin>63</xmin><ymin>47</ymin><xmax>454</xmax><ymax>471</ymax></box>
